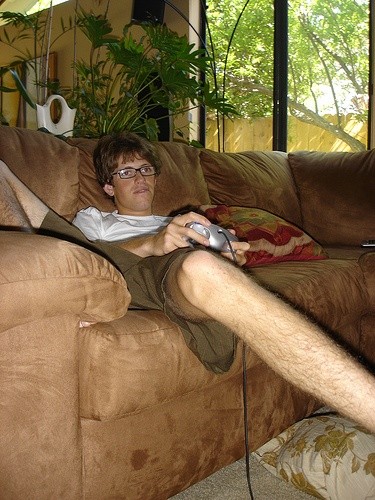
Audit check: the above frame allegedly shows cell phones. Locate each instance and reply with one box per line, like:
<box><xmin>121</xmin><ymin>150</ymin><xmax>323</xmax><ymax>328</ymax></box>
<box><xmin>360</xmin><ymin>240</ymin><xmax>375</xmax><ymax>248</ymax></box>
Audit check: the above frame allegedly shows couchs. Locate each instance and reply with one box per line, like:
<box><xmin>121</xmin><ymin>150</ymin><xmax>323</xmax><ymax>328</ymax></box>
<box><xmin>0</xmin><ymin>129</ymin><xmax>375</xmax><ymax>500</ymax></box>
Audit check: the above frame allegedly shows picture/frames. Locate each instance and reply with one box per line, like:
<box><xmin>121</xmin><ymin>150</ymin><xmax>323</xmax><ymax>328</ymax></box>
<box><xmin>20</xmin><ymin>52</ymin><xmax>59</xmax><ymax>129</ymax></box>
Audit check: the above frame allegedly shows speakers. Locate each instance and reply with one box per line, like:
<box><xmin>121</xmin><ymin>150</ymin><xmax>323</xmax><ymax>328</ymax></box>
<box><xmin>131</xmin><ymin>0</ymin><xmax>165</xmax><ymax>25</ymax></box>
<box><xmin>138</xmin><ymin>72</ymin><xmax>173</xmax><ymax>142</ymax></box>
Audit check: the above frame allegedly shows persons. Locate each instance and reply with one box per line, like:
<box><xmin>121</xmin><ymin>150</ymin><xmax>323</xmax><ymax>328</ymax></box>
<box><xmin>0</xmin><ymin>130</ymin><xmax>375</xmax><ymax>437</ymax></box>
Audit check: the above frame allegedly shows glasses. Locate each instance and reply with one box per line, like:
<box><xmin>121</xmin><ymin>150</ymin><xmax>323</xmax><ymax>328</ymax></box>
<box><xmin>112</xmin><ymin>166</ymin><xmax>156</xmax><ymax>179</ymax></box>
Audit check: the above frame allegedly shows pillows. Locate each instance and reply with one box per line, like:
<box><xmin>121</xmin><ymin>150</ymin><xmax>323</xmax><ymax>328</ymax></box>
<box><xmin>251</xmin><ymin>416</ymin><xmax>375</xmax><ymax>500</ymax></box>
<box><xmin>201</xmin><ymin>205</ymin><xmax>329</xmax><ymax>268</ymax></box>
